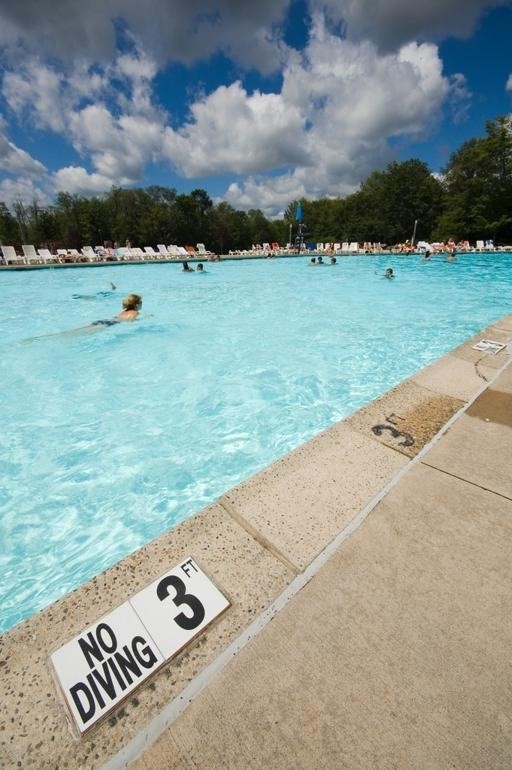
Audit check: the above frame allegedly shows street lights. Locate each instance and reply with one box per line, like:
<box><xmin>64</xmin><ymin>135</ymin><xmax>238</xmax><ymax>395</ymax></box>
<box><xmin>412</xmin><ymin>220</ymin><xmax>417</xmax><ymax>241</ymax></box>
<box><xmin>289</xmin><ymin>225</ymin><xmax>292</xmax><ymax>244</ymax></box>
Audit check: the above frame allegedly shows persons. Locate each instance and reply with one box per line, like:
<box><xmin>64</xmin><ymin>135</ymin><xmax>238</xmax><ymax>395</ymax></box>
<box><xmin>186</xmin><ymin>246</ymin><xmax>199</xmax><ymax>257</ymax></box>
<box><xmin>180</xmin><ymin>260</ymin><xmax>194</xmax><ymax>273</ymax></box>
<box><xmin>214</xmin><ymin>255</ymin><xmax>221</xmax><ymax>262</ymax></box>
<box><xmin>374</xmin><ymin>266</ymin><xmax>396</xmax><ymax>280</ymax></box>
<box><xmin>316</xmin><ymin>256</ymin><xmax>324</xmax><ymax>265</ymax></box>
<box><xmin>207</xmin><ymin>254</ymin><xmax>215</xmax><ymax>261</ymax></box>
<box><xmin>263</xmin><ymin>239</ymin><xmax>335</xmax><ymax>261</ymax></box>
<box><xmin>194</xmin><ymin>263</ymin><xmax>207</xmax><ymax>274</ymax></box>
<box><xmin>309</xmin><ymin>258</ymin><xmax>318</xmax><ymax>266</ymax></box>
<box><xmin>53</xmin><ymin>236</ymin><xmax>131</xmax><ymax>264</ymax></box>
<box><xmin>15</xmin><ymin>291</ymin><xmax>155</xmax><ymax>349</ymax></box>
<box><xmin>330</xmin><ymin>258</ymin><xmax>336</xmax><ymax>264</ymax></box>
<box><xmin>364</xmin><ymin>237</ymin><xmax>471</xmax><ymax>264</ymax></box>
<box><xmin>70</xmin><ymin>281</ymin><xmax>118</xmax><ymax>302</ymax></box>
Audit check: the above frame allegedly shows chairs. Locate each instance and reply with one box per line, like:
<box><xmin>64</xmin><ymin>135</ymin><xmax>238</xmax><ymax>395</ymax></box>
<box><xmin>475</xmin><ymin>239</ymin><xmax>496</xmax><ymax>252</ymax></box>
<box><xmin>229</xmin><ymin>241</ymin><xmax>382</xmax><ymax>256</ymax></box>
<box><xmin>1</xmin><ymin>243</ymin><xmax>216</xmax><ymax>265</ymax></box>
